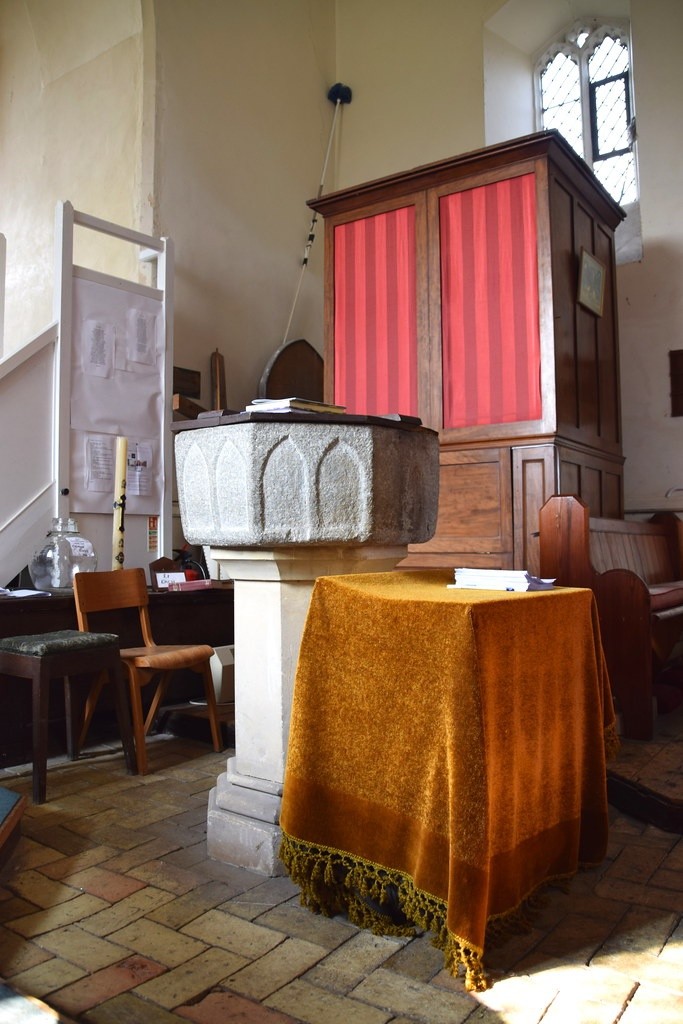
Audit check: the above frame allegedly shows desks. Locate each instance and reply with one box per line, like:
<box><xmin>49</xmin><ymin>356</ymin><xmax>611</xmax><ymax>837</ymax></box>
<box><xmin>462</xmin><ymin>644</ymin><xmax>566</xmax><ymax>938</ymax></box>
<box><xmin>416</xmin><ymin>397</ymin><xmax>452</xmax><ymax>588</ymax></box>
<box><xmin>278</xmin><ymin>567</ymin><xmax>621</xmax><ymax>990</ymax></box>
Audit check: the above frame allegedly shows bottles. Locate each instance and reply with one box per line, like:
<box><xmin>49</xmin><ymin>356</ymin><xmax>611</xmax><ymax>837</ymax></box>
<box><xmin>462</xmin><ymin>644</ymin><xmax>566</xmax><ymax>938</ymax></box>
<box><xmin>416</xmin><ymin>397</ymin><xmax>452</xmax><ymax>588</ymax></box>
<box><xmin>28</xmin><ymin>517</ymin><xmax>98</xmax><ymax>594</ymax></box>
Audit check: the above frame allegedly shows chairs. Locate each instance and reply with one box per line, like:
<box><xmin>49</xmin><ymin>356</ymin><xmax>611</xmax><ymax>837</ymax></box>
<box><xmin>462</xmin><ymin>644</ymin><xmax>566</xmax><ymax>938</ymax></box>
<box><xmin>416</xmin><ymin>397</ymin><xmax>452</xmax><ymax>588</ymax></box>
<box><xmin>73</xmin><ymin>568</ymin><xmax>224</xmax><ymax>776</ymax></box>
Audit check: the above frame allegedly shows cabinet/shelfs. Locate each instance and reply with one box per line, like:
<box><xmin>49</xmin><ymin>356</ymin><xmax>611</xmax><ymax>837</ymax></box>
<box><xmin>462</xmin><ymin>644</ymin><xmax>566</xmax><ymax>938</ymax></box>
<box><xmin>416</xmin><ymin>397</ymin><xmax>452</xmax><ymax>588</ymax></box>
<box><xmin>306</xmin><ymin>127</ymin><xmax>626</xmax><ymax>578</ymax></box>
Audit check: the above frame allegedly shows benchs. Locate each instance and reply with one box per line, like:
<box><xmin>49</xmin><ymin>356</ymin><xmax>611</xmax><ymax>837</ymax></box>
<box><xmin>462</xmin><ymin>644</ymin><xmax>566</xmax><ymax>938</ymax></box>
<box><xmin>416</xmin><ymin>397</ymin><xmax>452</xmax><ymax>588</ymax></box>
<box><xmin>537</xmin><ymin>495</ymin><xmax>683</xmax><ymax>741</ymax></box>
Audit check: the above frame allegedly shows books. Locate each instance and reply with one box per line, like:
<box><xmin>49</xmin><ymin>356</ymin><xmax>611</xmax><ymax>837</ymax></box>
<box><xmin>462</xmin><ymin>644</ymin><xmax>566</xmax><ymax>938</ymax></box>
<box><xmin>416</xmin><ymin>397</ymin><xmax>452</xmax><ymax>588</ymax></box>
<box><xmin>245</xmin><ymin>397</ymin><xmax>347</xmax><ymax>414</ymax></box>
<box><xmin>447</xmin><ymin>568</ymin><xmax>556</xmax><ymax>591</ymax></box>
<box><xmin>168</xmin><ymin>579</ymin><xmax>234</xmax><ymax>591</ymax></box>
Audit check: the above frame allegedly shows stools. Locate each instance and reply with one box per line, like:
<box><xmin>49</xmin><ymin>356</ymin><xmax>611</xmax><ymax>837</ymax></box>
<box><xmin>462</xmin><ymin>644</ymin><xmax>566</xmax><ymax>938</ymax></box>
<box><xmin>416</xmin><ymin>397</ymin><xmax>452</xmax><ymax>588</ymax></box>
<box><xmin>0</xmin><ymin>630</ymin><xmax>138</xmax><ymax>804</ymax></box>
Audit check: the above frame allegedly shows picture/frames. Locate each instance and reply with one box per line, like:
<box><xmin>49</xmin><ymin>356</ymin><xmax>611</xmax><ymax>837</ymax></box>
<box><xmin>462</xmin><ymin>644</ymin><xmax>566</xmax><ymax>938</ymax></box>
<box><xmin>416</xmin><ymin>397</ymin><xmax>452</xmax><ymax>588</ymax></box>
<box><xmin>576</xmin><ymin>246</ymin><xmax>607</xmax><ymax>318</ymax></box>
